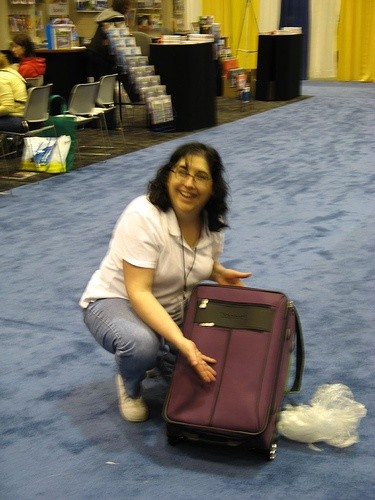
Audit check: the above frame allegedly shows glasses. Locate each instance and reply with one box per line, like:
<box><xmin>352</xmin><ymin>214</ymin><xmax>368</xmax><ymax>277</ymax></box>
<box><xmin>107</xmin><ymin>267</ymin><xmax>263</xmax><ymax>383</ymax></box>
<box><xmin>169</xmin><ymin>168</ymin><xmax>210</xmax><ymax>183</ymax></box>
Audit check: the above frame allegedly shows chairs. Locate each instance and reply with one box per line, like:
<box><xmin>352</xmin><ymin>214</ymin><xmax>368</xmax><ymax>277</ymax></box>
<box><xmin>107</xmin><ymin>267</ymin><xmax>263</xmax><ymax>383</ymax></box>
<box><xmin>85</xmin><ymin>73</ymin><xmax>125</xmax><ymax>147</ymax></box>
<box><xmin>53</xmin><ymin>81</ymin><xmax>108</xmax><ymax>170</ymax></box>
<box><xmin>0</xmin><ymin>84</ymin><xmax>64</xmax><ymax>185</ymax></box>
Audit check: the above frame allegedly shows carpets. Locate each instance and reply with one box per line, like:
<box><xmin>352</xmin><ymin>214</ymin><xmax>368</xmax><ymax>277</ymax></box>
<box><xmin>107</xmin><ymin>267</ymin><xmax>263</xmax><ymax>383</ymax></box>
<box><xmin>0</xmin><ymin>81</ymin><xmax>314</xmax><ymax>193</ymax></box>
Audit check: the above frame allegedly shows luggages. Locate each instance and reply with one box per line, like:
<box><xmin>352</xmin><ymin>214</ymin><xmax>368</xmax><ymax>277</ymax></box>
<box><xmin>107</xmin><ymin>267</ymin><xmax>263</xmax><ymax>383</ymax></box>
<box><xmin>164</xmin><ymin>282</ymin><xmax>304</xmax><ymax>460</ymax></box>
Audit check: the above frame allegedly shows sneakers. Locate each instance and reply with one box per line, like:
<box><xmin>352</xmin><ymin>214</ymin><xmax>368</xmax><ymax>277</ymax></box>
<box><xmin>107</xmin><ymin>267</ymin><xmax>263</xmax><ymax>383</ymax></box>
<box><xmin>116</xmin><ymin>374</ymin><xmax>148</xmax><ymax>422</ymax></box>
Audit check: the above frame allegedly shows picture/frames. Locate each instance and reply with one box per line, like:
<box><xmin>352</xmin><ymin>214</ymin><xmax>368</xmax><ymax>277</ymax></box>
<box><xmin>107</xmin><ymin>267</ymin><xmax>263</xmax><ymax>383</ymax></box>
<box><xmin>9</xmin><ymin>15</ymin><xmax>31</xmax><ymax>33</ymax></box>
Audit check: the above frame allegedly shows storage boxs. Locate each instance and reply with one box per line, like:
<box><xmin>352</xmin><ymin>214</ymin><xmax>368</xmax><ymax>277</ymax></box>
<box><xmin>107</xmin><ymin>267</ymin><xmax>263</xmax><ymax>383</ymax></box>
<box><xmin>222</xmin><ymin>58</ymin><xmax>239</xmax><ymax>81</ymax></box>
<box><xmin>120</xmin><ymin>103</ymin><xmax>149</xmax><ymax>127</ymax></box>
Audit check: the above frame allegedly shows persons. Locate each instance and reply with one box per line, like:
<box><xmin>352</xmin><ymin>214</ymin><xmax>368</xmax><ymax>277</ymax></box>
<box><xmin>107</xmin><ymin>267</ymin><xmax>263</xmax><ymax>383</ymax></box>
<box><xmin>80</xmin><ymin>140</ymin><xmax>251</xmax><ymax>424</ymax></box>
<box><xmin>12</xmin><ymin>33</ymin><xmax>49</xmax><ymax>80</ymax></box>
<box><xmin>0</xmin><ymin>53</ymin><xmax>30</xmax><ymax>128</ymax></box>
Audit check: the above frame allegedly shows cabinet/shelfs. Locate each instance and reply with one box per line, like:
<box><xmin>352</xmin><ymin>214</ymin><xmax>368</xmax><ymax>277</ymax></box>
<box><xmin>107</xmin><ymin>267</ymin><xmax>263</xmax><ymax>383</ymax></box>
<box><xmin>164</xmin><ymin>0</ymin><xmax>190</xmax><ymax>35</ymax></box>
<box><xmin>1</xmin><ymin>0</ymin><xmax>70</xmax><ymax>51</ymax></box>
<box><xmin>30</xmin><ymin>45</ymin><xmax>88</xmax><ymax>116</ymax></box>
<box><xmin>74</xmin><ymin>0</ymin><xmax>164</xmax><ymax>29</ymax></box>
<box><xmin>147</xmin><ymin>42</ymin><xmax>216</xmax><ymax>133</ymax></box>
<box><xmin>255</xmin><ymin>34</ymin><xmax>304</xmax><ymax>101</ymax></box>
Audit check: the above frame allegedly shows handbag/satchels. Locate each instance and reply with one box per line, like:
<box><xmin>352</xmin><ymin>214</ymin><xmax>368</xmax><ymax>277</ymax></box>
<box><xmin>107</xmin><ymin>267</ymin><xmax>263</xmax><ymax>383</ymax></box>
<box><xmin>48</xmin><ymin>116</ymin><xmax>76</xmax><ymax>170</ymax></box>
<box><xmin>19</xmin><ymin>135</ymin><xmax>71</xmax><ymax>174</ymax></box>
